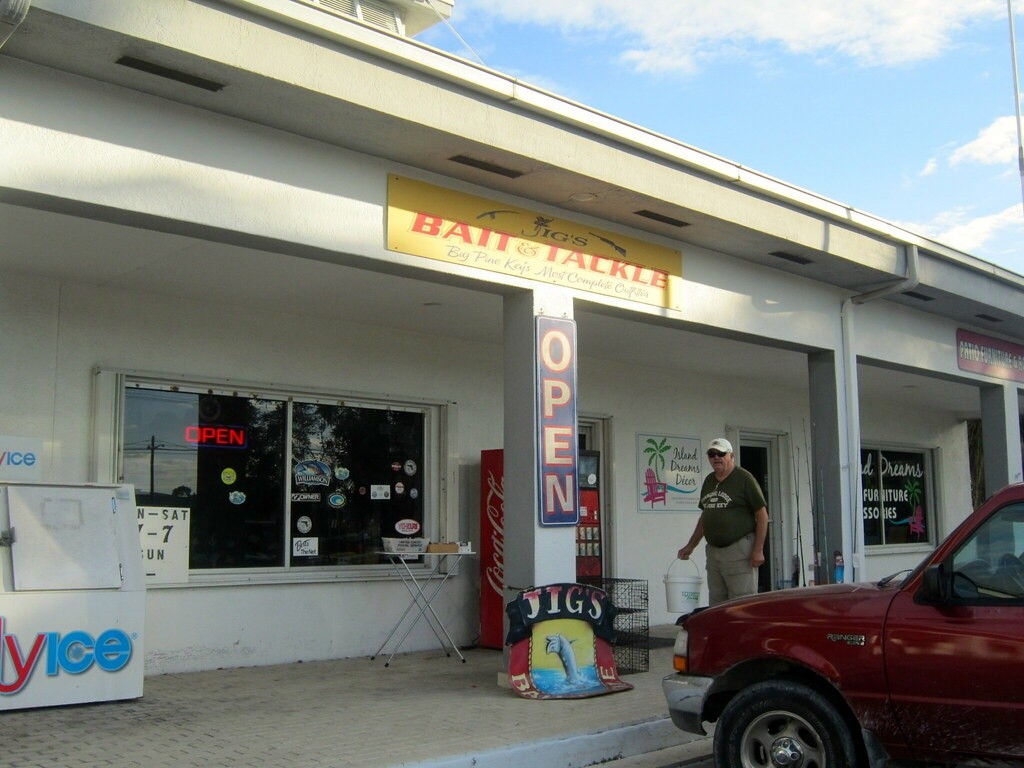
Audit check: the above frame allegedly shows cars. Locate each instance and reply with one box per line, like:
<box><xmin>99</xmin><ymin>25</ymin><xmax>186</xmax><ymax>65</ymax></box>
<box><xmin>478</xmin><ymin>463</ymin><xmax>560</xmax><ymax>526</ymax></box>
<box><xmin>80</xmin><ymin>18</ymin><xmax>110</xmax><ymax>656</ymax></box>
<box><xmin>661</xmin><ymin>481</ymin><xmax>1024</xmax><ymax>768</ymax></box>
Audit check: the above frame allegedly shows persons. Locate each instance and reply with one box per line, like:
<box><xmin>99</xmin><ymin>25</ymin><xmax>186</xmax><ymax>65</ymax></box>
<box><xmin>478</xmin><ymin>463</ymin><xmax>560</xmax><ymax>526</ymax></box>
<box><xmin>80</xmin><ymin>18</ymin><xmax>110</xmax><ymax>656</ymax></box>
<box><xmin>678</xmin><ymin>438</ymin><xmax>769</xmax><ymax>606</ymax></box>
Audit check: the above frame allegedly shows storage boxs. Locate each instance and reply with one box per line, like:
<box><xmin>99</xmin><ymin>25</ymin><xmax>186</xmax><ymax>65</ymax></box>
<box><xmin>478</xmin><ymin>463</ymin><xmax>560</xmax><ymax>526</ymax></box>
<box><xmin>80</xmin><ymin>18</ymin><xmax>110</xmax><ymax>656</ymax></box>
<box><xmin>456</xmin><ymin>541</ymin><xmax>472</xmax><ymax>552</ymax></box>
<box><xmin>381</xmin><ymin>537</ymin><xmax>431</xmax><ymax>553</ymax></box>
<box><xmin>427</xmin><ymin>544</ymin><xmax>458</xmax><ymax>553</ymax></box>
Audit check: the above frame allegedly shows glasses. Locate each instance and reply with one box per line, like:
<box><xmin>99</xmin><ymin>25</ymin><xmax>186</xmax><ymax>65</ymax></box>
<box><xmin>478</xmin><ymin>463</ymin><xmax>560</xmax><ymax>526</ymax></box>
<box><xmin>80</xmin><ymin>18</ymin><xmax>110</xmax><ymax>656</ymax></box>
<box><xmin>709</xmin><ymin>451</ymin><xmax>727</xmax><ymax>457</ymax></box>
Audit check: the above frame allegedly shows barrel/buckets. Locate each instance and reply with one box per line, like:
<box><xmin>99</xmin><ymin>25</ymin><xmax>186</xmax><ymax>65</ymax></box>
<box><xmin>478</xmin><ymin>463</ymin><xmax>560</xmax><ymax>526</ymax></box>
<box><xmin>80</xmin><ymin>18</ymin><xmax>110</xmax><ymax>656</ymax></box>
<box><xmin>663</xmin><ymin>556</ymin><xmax>702</xmax><ymax>613</ymax></box>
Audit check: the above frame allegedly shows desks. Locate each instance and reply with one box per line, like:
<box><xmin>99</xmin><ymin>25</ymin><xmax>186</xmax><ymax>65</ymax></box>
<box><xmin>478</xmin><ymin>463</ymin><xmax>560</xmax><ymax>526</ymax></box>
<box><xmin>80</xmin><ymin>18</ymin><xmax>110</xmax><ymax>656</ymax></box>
<box><xmin>370</xmin><ymin>549</ymin><xmax>477</xmax><ymax>668</ymax></box>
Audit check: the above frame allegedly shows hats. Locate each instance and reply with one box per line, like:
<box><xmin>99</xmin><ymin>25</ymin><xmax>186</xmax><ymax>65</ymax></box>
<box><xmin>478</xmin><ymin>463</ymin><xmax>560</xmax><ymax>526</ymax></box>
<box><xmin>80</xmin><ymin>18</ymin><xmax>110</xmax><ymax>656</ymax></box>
<box><xmin>707</xmin><ymin>438</ymin><xmax>733</xmax><ymax>453</ymax></box>
